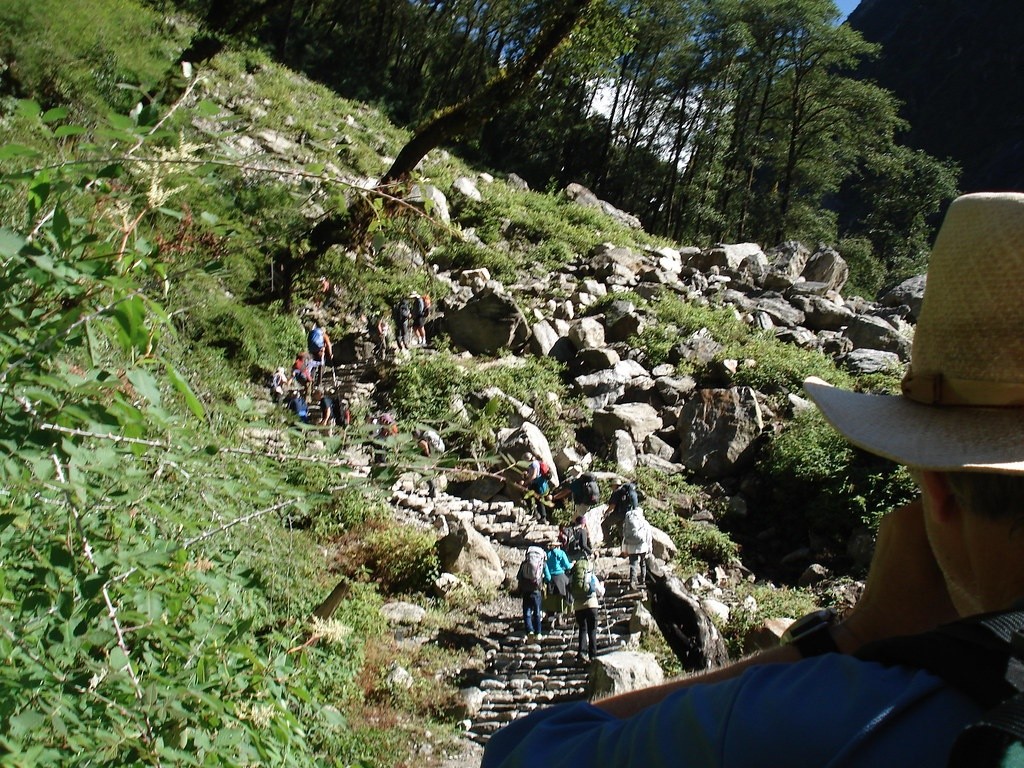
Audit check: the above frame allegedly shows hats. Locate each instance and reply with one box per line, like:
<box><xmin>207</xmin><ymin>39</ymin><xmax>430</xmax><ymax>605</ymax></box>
<box><xmin>608</xmin><ymin>479</ymin><xmax>622</xmax><ymax>486</ymax></box>
<box><xmin>408</xmin><ymin>290</ymin><xmax>423</xmax><ymax>299</ymax></box>
<box><xmin>800</xmin><ymin>188</ymin><xmax>1024</xmax><ymax>480</ymax></box>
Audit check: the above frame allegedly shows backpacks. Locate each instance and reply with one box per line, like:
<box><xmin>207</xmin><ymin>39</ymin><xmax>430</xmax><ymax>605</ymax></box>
<box><xmin>624</xmin><ymin>510</ymin><xmax>647</xmax><ymax>545</ymax></box>
<box><xmin>539</xmin><ymin>461</ymin><xmax>553</xmax><ymax>481</ymax></box>
<box><xmin>584</xmin><ymin>480</ymin><xmax>600</xmax><ymax>505</ymax></box>
<box><xmin>380</xmin><ymin>414</ymin><xmax>400</xmax><ymax>438</ymax></box>
<box><xmin>329</xmin><ymin>385</ymin><xmax>345</xmax><ymax>426</ymax></box>
<box><xmin>307</xmin><ymin>328</ymin><xmax>325</xmax><ymax>356</ymax></box>
<box><xmin>522</xmin><ymin>544</ymin><xmax>549</xmax><ymax>582</ymax></box>
<box><xmin>568</xmin><ymin>559</ymin><xmax>595</xmax><ymax>603</ymax></box>
<box><xmin>422</xmin><ymin>429</ymin><xmax>446</xmax><ymax>453</ymax></box>
<box><xmin>422</xmin><ymin>295</ymin><xmax>430</xmax><ymax>318</ymax></box>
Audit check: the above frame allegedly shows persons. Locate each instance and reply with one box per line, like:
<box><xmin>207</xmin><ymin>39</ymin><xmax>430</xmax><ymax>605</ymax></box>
<box><xmin>272</xmin><ymin>289</ymin><xmax>445</xmax><ymax>498</ymax></box>
<box><xmin>481</xmin><ymin>194</ymin><xmax>1024</xmax><ymax>768</ymax></box>
<box><xmin>517</xmin><ymin>452</ymin><xmax>653</xmax><ymax>665</ymax></box>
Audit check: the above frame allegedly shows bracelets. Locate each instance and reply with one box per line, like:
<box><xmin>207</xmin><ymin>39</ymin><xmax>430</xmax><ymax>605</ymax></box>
<box><xmin>779</xmin><ymin>606</ymin><xmax>843</xmax><ymax>661</ymax></box>
